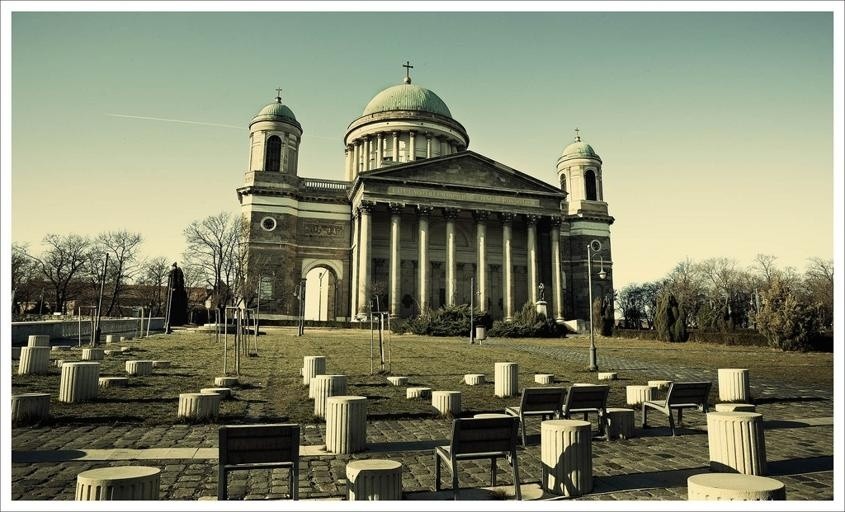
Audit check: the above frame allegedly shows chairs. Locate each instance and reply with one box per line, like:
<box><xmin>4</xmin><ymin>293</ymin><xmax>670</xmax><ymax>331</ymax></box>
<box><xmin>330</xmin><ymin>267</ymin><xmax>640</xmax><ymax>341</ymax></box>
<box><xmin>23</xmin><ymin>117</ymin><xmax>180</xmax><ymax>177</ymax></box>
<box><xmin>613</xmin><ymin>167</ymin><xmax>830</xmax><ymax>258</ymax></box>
<box><xmin>561</xmin><ymin>384</ymin><xmax>610</xmax><ymax>442</ymax></box>
<box><xmin>505</xmin><ymin>387</ymin><xmax>565</xmax><ymax>449</ymax></box>
<box><xmin>218</xmin><ymin>424</ymin><xmax>300</xmax><ymax>500</ymax></box>
<box><xmin>435</xmin><ymin>416</ymin><xmax>522</xmax><ymax>499</ymax></box>
<box><xmin>642</xmin><ymin>381</ymin><xmax>712</xmax><ymax>437</ymax></box>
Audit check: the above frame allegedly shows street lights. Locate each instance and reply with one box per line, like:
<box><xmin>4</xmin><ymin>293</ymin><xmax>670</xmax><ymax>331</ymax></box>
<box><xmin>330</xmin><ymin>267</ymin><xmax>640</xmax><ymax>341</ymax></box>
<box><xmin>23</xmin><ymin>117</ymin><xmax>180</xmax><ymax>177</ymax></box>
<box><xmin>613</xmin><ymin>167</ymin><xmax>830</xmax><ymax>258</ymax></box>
<box><xmin>470</xmin><ymin>277</ymin><xmax>483</xmax><ymax>343</ymax></box>
<box><xmin>586</xmin><ymin>245</ymin><xmax>607</xmax><ymax>373</ymax></box>
<box><xmin>295</xmin><ymin>280</ymin><xmax>305</xmax><ymax>337</ymax></box>
